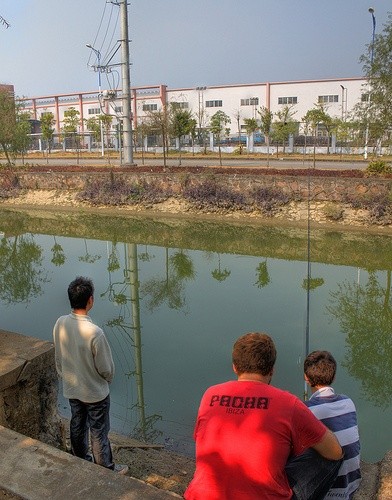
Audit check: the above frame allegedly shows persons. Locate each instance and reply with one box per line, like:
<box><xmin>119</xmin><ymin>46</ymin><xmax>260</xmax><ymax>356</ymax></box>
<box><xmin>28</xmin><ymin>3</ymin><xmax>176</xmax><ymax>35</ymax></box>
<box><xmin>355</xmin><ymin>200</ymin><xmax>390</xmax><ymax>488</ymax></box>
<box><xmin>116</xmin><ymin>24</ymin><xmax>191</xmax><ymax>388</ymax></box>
<box><xmin>183</xmin><ymin>332</ymin><xmax>344</xmax><ymax>500</ymax></box>
<box><xmin>300</xmin><ymin>350</ymin><xmax>360</xmax><ymax>500</ymax></box>
<box><xmin>55</xmin><ymin>276</ymin><xmax>129</xmax><ymax>474</ymax></box>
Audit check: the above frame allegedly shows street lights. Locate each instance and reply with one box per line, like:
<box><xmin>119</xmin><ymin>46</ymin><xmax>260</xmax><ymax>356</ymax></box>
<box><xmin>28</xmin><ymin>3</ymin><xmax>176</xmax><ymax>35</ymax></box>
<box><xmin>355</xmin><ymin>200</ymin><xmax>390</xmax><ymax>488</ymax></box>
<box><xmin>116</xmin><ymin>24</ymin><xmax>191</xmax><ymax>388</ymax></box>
<box><xmin>85</xmin><ymin>43</ymin><xmax>107</xmax><ymax>157</ymax></box>
<box><xmin>99</xmin><ymin>240</ymin><xmax>119</xmax><ymax>301</ymax></box>
<box><xmin>363</xmin><ymin>7</ymin><xmax>377</xmax><ymax>160</ymax></box>
<box><xmin>347</xmin><ymin>267</ymin><xmax>361</xmax><ymax>337</ymax></box>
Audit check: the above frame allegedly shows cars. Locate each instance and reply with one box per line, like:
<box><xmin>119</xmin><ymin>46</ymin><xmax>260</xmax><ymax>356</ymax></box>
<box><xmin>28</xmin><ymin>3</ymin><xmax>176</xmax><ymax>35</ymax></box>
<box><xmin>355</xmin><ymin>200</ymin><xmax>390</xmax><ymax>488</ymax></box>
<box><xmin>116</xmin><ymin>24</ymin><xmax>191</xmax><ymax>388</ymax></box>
<box><xmin>294</xmin><ymin>135</ymin><xmax>331</xmax><ymax>146</ymax></box>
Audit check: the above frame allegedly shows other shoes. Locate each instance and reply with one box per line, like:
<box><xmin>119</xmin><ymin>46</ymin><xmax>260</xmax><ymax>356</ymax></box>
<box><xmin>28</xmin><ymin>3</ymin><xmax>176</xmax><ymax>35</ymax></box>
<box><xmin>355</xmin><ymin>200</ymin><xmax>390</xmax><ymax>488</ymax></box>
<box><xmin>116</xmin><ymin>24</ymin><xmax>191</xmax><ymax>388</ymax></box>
<box><xmin>114</xmin><ymin>465</ymin><xmax>129</xmax><ymax>474</ymax></box>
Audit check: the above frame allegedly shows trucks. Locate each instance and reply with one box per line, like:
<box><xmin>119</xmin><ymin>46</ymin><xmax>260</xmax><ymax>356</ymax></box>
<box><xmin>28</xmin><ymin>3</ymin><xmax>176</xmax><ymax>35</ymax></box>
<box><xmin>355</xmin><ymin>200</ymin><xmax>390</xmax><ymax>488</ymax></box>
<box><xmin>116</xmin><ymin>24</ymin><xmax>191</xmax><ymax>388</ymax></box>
<box><xmin>232</xmin><ymin>133</ymin><xmax>265</xmax><ymax>144</ymax></box>
